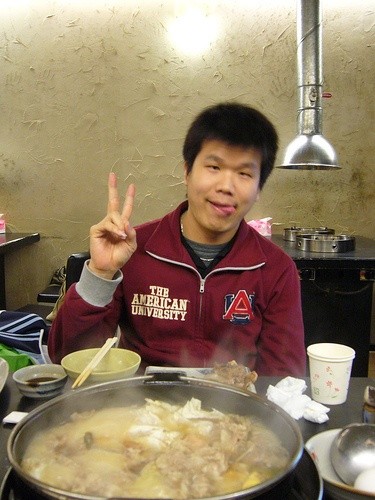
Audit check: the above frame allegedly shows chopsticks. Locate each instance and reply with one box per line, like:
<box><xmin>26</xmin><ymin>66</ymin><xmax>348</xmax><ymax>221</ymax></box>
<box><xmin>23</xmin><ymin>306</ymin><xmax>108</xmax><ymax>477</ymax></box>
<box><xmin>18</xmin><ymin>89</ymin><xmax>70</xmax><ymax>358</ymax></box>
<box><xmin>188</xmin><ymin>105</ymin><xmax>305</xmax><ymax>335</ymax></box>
<box><xmin>71</xmin><ymin>336</ymin><xmax>118</xmax><ymax>388</ymax></box>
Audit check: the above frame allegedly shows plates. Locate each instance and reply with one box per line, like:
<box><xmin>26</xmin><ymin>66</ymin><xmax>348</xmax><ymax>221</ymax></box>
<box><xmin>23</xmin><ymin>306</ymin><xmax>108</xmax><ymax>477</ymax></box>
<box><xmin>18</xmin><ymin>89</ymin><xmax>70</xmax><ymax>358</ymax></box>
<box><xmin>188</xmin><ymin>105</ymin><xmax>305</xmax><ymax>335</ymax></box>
<box><xmin>145</xmin><ymin>365</ymin><xmax>256</xmax><ymax>394</ymax></box>
<box><xmin>0</xmin><ymin>357</ymin><xmax>9</xmax><ymax>393</ymax></box>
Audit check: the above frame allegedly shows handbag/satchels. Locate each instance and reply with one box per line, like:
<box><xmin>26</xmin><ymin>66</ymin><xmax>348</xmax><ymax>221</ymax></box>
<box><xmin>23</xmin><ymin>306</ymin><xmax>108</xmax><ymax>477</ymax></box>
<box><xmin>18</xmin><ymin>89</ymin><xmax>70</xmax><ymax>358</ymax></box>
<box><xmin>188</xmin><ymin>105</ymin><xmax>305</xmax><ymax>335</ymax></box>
<box><xmin>44</xmin><ymin>266</ymin><xmax>66</xmax><ymax>321</ymax></box>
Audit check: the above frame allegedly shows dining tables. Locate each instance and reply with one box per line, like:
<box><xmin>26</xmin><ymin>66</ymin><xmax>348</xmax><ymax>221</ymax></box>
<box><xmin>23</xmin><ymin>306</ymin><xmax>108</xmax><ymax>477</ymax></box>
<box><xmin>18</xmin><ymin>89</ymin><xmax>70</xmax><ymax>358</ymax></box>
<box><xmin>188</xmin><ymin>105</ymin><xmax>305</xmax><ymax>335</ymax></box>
<box><xmin>0</xmin><ymin>369</ymin><xmax>375</xmax><ymax>500</ymax></box>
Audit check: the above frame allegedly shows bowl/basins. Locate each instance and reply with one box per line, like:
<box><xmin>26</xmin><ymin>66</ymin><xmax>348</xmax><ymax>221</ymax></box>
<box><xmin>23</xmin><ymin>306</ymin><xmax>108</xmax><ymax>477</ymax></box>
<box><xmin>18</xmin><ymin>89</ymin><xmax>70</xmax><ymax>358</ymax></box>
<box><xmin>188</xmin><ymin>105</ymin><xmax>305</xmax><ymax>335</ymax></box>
<box><xmin>304</xmin><ymin>428</ymin><xmax>375</xmax><ymax>500</ymax></box>
<box><xmin>61</xmin><ymin>348</ymin><xmax>141</xmax><ymax>389</ymax></box>
<box><xmin>12</xmin><ymin>364</ymin><xmax>68</xmax><ymax>399</ymax></box>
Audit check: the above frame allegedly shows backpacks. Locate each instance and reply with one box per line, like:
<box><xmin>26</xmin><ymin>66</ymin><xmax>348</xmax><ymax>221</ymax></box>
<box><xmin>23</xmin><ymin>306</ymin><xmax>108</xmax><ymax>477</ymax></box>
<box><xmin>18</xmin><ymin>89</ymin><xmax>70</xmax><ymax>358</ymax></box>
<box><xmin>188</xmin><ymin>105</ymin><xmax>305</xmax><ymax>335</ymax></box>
<box><xmin>0</xmin><ymin>309</ymin><xmax>49</xmax><ymax>354</ymax></box>
<box><xmin>0</xmin><ymin>343</ymin><xmax>40</xmax><ymax>371</ymax></box>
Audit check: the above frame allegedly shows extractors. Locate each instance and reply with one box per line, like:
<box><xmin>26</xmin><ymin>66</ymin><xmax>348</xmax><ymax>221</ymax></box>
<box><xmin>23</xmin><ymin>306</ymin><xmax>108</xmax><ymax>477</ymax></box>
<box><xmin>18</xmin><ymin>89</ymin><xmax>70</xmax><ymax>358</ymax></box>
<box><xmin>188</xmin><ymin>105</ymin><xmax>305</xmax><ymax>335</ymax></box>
<box><xmin>273</xmin><ymin>0</ymin><xmax>342</xmax><ymax>169</ymax></box>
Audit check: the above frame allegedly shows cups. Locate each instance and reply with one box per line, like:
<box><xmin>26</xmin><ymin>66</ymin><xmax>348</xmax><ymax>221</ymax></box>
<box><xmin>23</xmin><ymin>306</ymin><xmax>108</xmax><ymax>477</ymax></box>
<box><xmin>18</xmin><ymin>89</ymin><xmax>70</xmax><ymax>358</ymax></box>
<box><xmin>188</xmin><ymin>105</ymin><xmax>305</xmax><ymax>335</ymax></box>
<box><xmin>306</xmin><ymin>343</ymin><xmax>355</xmax><ymax>405</ymax></box>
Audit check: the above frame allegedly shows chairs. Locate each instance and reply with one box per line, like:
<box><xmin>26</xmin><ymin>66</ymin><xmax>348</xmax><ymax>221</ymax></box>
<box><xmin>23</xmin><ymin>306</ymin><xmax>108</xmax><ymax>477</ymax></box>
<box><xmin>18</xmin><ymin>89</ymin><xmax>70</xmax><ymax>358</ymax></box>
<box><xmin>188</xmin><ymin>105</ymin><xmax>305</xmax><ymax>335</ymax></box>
<box><xmin>15</xmin><ymin>252</ymin><xmax>90</xmax><ymax>327</ymax></box>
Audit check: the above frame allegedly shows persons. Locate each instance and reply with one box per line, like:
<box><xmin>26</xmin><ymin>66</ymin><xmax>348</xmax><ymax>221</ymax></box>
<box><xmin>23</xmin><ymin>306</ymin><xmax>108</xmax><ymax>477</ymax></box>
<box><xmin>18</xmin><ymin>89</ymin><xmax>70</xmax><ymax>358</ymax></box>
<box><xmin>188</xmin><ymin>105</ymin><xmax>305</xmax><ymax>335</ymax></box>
<box><xmin>48</xmin><ymin>102</ymin><xmax>305</xmax><ymax>378</ymax></box>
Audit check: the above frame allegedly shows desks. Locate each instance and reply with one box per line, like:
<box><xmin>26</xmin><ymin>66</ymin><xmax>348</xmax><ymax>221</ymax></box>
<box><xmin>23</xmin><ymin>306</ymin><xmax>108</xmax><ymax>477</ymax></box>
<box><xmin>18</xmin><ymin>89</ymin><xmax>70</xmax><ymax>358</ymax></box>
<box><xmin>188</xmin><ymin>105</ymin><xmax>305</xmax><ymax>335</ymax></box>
<box><xmin>0</xmin><ymin>232</ymin><xmax>40</xmax><ymax>311</ymax></box>
<box><xmin>271</xmin><ymin>233</ymin><xmax>375</xmax><ymax>377</ymax></box>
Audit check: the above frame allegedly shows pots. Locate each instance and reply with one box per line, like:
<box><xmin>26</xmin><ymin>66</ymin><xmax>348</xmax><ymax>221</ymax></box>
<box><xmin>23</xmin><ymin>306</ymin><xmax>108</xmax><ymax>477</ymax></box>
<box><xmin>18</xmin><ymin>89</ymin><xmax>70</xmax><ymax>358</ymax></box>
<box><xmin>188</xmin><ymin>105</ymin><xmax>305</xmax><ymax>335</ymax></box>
<box><xmin>6</xmin><ymin>371</ymin><xmax>303</xmax><ymax>500</ymax></box>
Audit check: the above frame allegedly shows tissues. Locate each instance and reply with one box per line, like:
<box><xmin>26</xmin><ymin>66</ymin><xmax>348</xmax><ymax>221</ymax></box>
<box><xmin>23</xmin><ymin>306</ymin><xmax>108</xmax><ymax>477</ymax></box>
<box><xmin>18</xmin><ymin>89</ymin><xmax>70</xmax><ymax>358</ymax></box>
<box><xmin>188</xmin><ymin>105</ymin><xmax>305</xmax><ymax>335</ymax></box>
<box><xmin>0</xmin><ymin>214</ymin><xmax>7</xmax><ymax>234</ymax></box>
<box><xmin>246</xmin><ymin>216</ymin><xmax>272</xmax><ymax>237</ymax></box>
